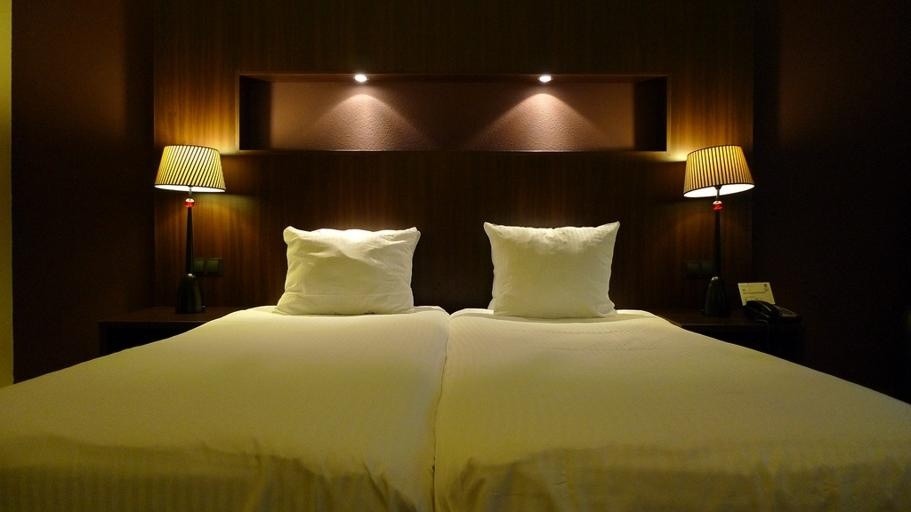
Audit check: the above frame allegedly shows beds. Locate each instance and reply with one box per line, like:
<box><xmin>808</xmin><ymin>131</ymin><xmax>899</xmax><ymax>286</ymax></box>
<box><xmin>0</xmin><ymin>147</ymin><xmax>911</xmax><ymax>511</ymax></box>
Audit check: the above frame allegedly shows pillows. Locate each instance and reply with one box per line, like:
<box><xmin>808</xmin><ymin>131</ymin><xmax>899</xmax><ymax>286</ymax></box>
<box><xmin>270</xmin><ymin>226</ymin><xmax>422</xmax><ymax>315</ymax></box>
<box><xmin>482</xmin><ymin>220</ymin><xmax>622</xmax><ymax>316</ymax></box>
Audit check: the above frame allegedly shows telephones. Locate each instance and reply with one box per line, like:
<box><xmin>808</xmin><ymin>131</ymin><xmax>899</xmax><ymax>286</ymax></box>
<box><xmin>745</xmin><ymin>300</ymin><xmax>800</xmax><ymax>322</ymax></box>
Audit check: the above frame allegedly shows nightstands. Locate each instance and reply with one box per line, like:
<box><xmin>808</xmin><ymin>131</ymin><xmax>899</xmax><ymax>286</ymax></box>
<box><xmin>98</xmin><ymin>306</ymin><xmax>237</xmax><ymax>359</ymax></box>
<box><xmin>647</xmin><ymin>303</ymin><xmax>801</xmax><ymax>365</ymax></box>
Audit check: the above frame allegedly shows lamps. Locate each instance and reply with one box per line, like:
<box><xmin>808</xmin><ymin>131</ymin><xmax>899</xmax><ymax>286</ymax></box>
<box><xmin>682</xmin><ymin>143</ymin><xmax>756</xmax><ymax>318</ymax></box>
<box><xmin>154</xmin><ymin>144</ymin><xmax>226</xmax><ymax>314</ymax></box>
